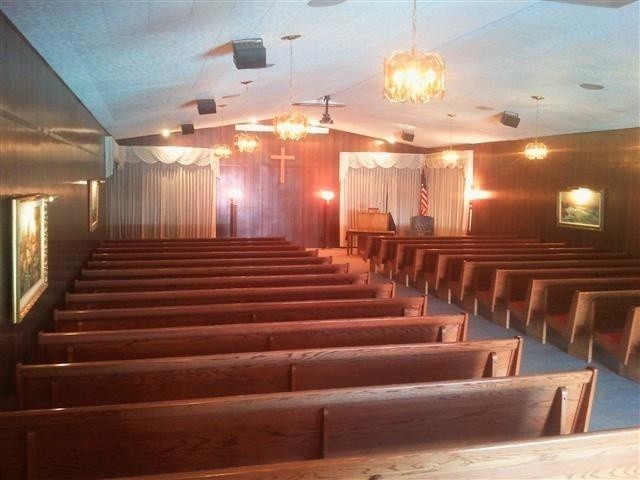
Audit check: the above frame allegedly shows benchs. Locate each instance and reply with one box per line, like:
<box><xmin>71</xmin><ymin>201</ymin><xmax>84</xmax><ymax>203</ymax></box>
<box><xmin>1</xmin><ymin>229</ymin><xmax>639</xmax><ymax>480</ymax></box>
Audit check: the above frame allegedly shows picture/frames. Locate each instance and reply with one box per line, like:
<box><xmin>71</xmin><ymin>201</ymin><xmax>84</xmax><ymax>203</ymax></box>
<box><xmin>11</xmin><ymin>190</ymin><xmax>50</xmax><ymax>326</ymax></box>
<box><xmin>555</xmin><ymin>182</ymin><xmax>606</xmax><ymax>231</ymax></box>
<box><xmin>87</xmin><ymin>176</ymin><xmax>100</xmax><ymax>232</ymax></box>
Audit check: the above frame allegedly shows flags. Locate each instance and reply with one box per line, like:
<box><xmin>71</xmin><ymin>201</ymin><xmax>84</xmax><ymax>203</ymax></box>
<box><xmin>419</xmin><ymin>168</ymin><xmax>429</xmax><ymax>216</ymax></box>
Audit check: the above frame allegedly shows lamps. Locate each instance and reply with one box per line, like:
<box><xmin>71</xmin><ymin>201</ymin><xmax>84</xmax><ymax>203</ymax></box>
<box><xmin>383</xmin><ymin>1</ymin><xmax>447</xmax><ymax>106</ymax></box>
<box><xmin>233</xmin><ymin>80</ymin><xmax>262</xmax><ymax>154</ymax></box>
<box><xmin>274</xmin><ymin>32</ymin><xmax>309</xmax><ymax>142</ymax></box>
<box><xmin>441</xmin><ymin>113</ymin><xmax>460</xmax><ymax>163</ymax></box>
<box><xmin>523</xmin><ymin>95</ymin><xmax>548</xmax><ymax>161</ymax></box>
<box><xmin>212</xmin><ymin>103</ymin><xmax>233</xmax><ymax>159</ymax></box>
